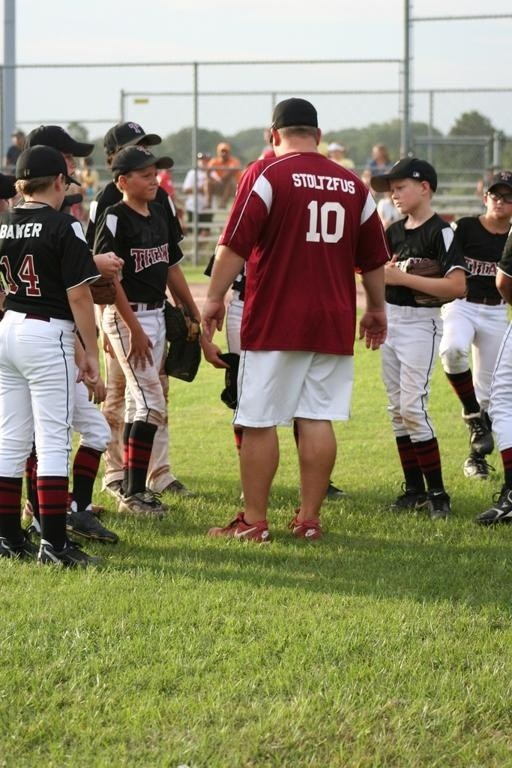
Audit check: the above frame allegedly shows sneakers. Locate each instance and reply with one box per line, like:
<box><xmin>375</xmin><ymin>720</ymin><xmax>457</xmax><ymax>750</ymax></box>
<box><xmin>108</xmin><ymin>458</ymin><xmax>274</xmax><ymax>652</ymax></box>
<box><xmin>65</xmin><ymin>511</ymin><xmax>118</xmax><ymax>544</ymax></box>
<box><xmin>70</xmin><ymin>501</ymin><xmax>104</xmax><ymax>514</ymax></box>
<box><xmin>287</xmin><ymin>509</ymin><xmax>321</xmax><ymax>539</ymax></box>
<box><xmin>427</xmin><ymin>489</ymin><xmax>450</xmax><ymax>520</ymax></box>
<box><xmin>462</xmin><ymin>407</ymin><xmax>494</xmax><ymax>455</ymax></box>
<box><xmin>209</xmin><ymin>512</ymin><xmax>272</xmax><ymax>544</ymax></box>
<box><xmin>474</xmin><ymin>484</ymin><xmax>512</xmax><ymax>524</ymax></box>
<box><xmin>106</xmin><ymin>480</ymin><xmax>123</xmax><ymax>494</ymax></box>
<box><xmin>392</xmin><ymin>482</ymin><xmax>427</xmax><ymax>509</ymax></box>
<box><xmin>0</xmin><ymin>537</ymin><xmax>39</xmax><ymax>562</ymax></box>
<box><xmin>322</xmin><ymin>479</ymin><xmax>352</xmax><ymax>501</ymax></box>
<box><xmin>40</xmin><ymin>537</ymin><xmax>100</xmax><ymax>570</ymax></box>
<box><xmin>23</xmin><ymin>500</ymin><xmax>34</xmax><ymax>520</ymax></box>
<box><xmin>162</xmin><ymin>480</ymin><xmax>196</xmax><ymax>498</ymax></box>
<box><xmin>27</xmin><ymin>516</ymin><xmax>41</xmax><ymax>538</ymax></box>
<box><xmin>118</xmin><ymin>493</ymin><xmax>169</xmax><ymax>513</ymax></box>
<box><xmin>463</xmin><ymin>452</ymin><xmax>496</xmax><ymax>479</ymax></box>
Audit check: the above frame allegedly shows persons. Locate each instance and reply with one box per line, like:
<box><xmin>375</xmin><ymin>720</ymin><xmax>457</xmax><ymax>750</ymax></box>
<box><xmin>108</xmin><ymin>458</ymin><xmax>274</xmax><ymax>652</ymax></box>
<box><xmin>182</xmin><ymin>152</ymin><xmax>211</xmax><ymax>262</ymax></box>
<box><xmin>327</xmin><ymin>142</ymin><xmax>357</xmax><ymax>177</ymax></box>
<box><xmin>203</xmin><ymin>256</ymin><xmax>348</xmax><ymax>505</ymax></box>
<box><xmin>92</xmin><ymin>144</ymin><xmax>172</xmax><ymax>517</ymax></box>
<box><xmin>199</xmin><ymin>139</ymin><xmax>245</xmax><ymax>212</ymax></box>
<box><xmin>26</xmin><ymin>194</ymin><xmax>119</xmax><ymax>549</ymax></box>
<box><xmin>199</xmin><ymin>95</ymin><xmax>389</xmax><ymax>545</ymax></box>
<box><xmin>26</xmin><ymin>128</ymin><xmax>95</xmax><ymax>177</ymax></box>
<box><xmin>360</xmin><ymin>144</ymin><xmax>390</xmax><ymax>204</ymax></box>
<box><xmin>87</xmin><ymin>123</ymin><xmax>195</xmax><ymax>501</ymax></box>
<box><xmin>80</xmin><ymin>170</ymin><xmax>104</xmax><ymax>204</ymax></box>
<box><xmin>441</xmin><ymin>169</ymin><xmax>512</xmax><ymax>482</ymax></box>
<box><xmin>470</xmin><ymin>218</ymin><xmax>512</xmax><ymax>527</ymax></box>
<box><xmin>0</xmin><ymin>143</ymin><xmax>106</xmax><ymax>570</ymax></box>
<box><xmin>94</xmin><ymin>146</ymin><xmax>203</xmax><ymax>518</ymax></box>
<box><xmin>147</xmin><ymin>166</ymin><xmax>185</xmax><ymax>225</ymax></box>
<box><xmin>377</xmin><ymin>154</ymin><xmax>468</xmax><ymax>512</ymax></box>
<box><xmin>5</xmin><ymin>129</ymin><xmax>27</xmax><ymax>146</ymax></box>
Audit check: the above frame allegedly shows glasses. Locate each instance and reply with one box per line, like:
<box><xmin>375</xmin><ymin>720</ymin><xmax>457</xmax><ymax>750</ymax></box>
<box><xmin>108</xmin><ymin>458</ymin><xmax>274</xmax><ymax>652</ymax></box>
<box><xmin>487</xmin><ymin>190</ymin><xmax>512</xmax><ymax>204</ymax></box>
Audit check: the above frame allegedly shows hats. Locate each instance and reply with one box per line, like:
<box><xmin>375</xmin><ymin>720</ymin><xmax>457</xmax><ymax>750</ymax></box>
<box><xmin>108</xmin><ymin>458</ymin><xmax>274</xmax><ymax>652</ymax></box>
<box><xmin>218</xmin><ymin>353</ymin><xmax>240</xmax><ymax>409</ymax></box>
<box><xmin>483</xmin><ymin>170</ymin><xmax>512</xmax><ymax>192</ymax></box>
<box><xmin>0</xmin><ymin>172</ymin><xmax>18</xmax><ymax>199</ymax></box>
<box><xmin>327</xmin><ymin>142</ymin><xmax>345</xmax><ymax>153</ymax></box>
<box><xmin>16</xmin><ymin>146</ymin><xmax>82</xmax><ymax>187</ymax></box>
<box><xmin>24</xmin><ymin>125</ymin><xmax>95</xmax><ymax>157</ymax></box>
<box><xmin>371</xmin><ymin>158</ymin><xmax>437</xmax><ymax>192</ymax></box>
<box><xmin>270</xmin><ymin>98</ymin><xmax>318</xmax><ymax>142</ymax></box>
<box><xmin>112</xmin><ymin>145</ymin><xmax>175</xmax><ymax>183</ymax></box>
<box><xmin>60</xmin><ymin>193</ymin><xmax>83</xmax><ymax>212</ymax></box>
<box><xmin>104</xmin><ymin>121</ymin><xmax>162</xmax><ymax>163</ymax></box>
